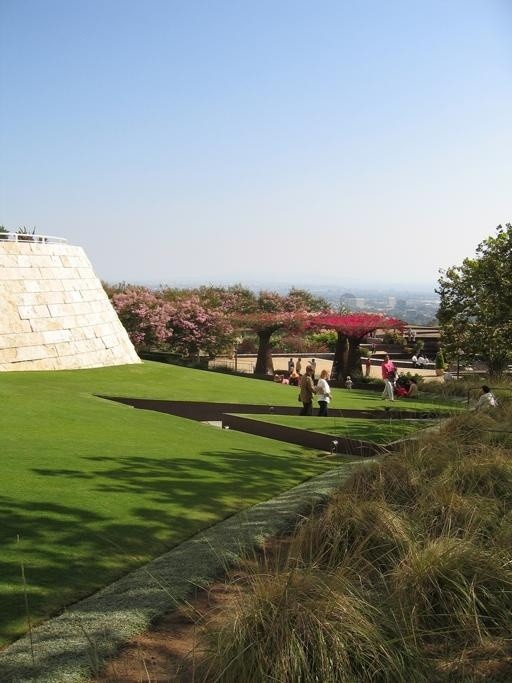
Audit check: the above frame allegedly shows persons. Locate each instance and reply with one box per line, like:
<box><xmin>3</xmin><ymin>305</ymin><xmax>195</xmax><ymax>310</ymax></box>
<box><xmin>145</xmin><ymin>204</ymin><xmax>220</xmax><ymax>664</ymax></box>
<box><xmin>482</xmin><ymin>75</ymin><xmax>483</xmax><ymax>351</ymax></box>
<box><xmin>344</xmin><ymin>375</ymin><xmax>354</xmax><ymax>391</ymax></box>
<box><xmin>389</xmin><ymin>374</ymin><xmax>419</xmax><ymax>399</ymax></box>
<box><xmin>365</xmin><ymin>354</ymin><xmax>371</xmax><ymax>377</ymax></box>
<box><xmin>273</xmin><ymin>357</ymin><xmax>316</xmax><ymax>386</ymax></box>
<box><xmin>468</xmin><ymin>384</ymin><xmax>496</xmax><ymax>411</ymax></box>
<box><xmin>416</xmin><ymin>354</ymin><xmax>425</xmax><ymax>368</ymax></box>
<box><xmin>424</xmin><ymin>354</ymin><xmax>429</xmax><ymax>363</ymax></box>
<box><xmin>315</xmin><ymin>370</ymin><xmax>332</xmax><ymax>416</ymax></box>
<box><xmin>298</xmin><ymin>366</ymin><xmax>316</xmax><ymax>416</ymax></box>
<box><xmin>381</xmin><ymin>353</ymin><xmax>396</xmax><ymax>401</ymax></box>
<box><xmin>411</xmin><ymin>352</ymin><xmax>417</xmax><ymax>368</ymax></box>
